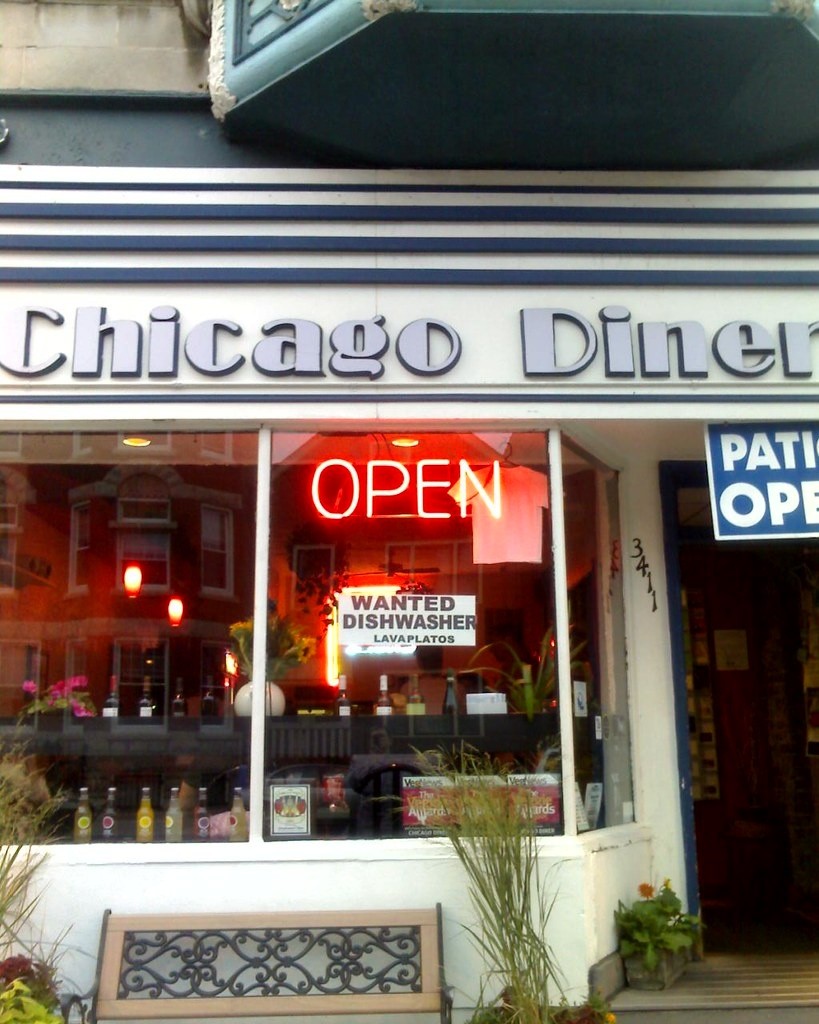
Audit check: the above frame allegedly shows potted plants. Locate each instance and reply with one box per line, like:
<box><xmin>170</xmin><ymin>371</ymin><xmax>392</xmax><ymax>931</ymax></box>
<box><xmin>412</xmin><ymin>740</ymin><xmax>617</xmax><ymax>1024</ymax></box>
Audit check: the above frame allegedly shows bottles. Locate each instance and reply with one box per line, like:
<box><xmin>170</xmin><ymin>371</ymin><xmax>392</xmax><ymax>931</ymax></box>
<box><xmin>73</xmin><ymin>787</ymin><xmax>92</xmax><ymax>843</ymax></box>
<box><xmin>193</xmin><ymin>788</ymin><xmax>211</xmax><ymax>840</ymax></box>
<box><xmin>473</xmin><ymin>673</ymin><xmax>486</xmax><ymax>693</ymax></box>
<box><xmin>372</xmin><ymin>674</ymin><xmax>396</xmax><ymax>716</ymax></box>
<box><xmin>334</xmin><ymin>673</ymin><xmax>354</xmax><ymax>717</ymax></box>
<box><xmin>406</xmin><ymin>674</ymin><xmax>426</xmax><ymax>716</ymax></box>
<box><xmin>135</xmin><ymin>788</ymin><xmax>154</xmax><ymax>843</ymax></box>
<box><xmin>101</xmin><ymin>676</ymin><xmax>121</xmax><ymax>718</ymax></box>
<box><xmin>101</xmin><ymin>787</ymin><xmax>118</xmax><ymax>843</ymax></box>
<box><xmin>138</xmin><ymin>676</ymin><xmax>158</xmax><ymax>717</ymax></box>
<box><xmin>441</xmin><ymin>669</ymin><xmax>460</xmax><ymax>715</ymax></box>
<box><xmin>229</xmin><ymin>786</ymin><xmax>248</xmax><ymax>843</ymax></box>
<box><xmin>200</xmin><ymin>676</ymin><xmax>219</xmax><ymax>718</ymax></box>
<box><xmin>164</xmin><ymin>787</ymin><xmax>182</xmax><ymax>843</ymax></box>
<box><xmin>171</xmin><ymin>678</ymin><xmax>189</xmax><ymax>718</ymax></box>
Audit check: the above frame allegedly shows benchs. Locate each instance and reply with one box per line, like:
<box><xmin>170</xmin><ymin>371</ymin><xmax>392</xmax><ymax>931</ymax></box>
<box><xmin>61</xmin><ymin>901</ymin><xmax>456</xmax><ymax>1024</ymax></box>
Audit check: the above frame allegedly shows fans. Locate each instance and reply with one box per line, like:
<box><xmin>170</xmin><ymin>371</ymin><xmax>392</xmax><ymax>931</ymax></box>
<box><xmin>615</xmin><ymin>877</ymin><xmax>705</xmax><ymax>969</ymax></box>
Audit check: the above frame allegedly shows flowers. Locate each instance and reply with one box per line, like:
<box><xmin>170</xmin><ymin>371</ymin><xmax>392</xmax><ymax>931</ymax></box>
<box><xmin>227</xmin><ymin>615</ymin><xmax>315</xmax><ymax>681</ymax></box>
<box><xmin>9</xmin><ymin>674</ymin><xmax>98</xmax><ymax>730</ymax></box>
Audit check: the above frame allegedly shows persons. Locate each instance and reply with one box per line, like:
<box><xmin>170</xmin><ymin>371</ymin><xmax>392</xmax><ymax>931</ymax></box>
<box><xmin>455</xmin><ymin>672</ymin><xmax>487</xmax><ymax>714</ymax></box>
<box><xmin>399</xmin><ymin>647</ymin><xmax>466</xmax><ymax>715</ymax></box>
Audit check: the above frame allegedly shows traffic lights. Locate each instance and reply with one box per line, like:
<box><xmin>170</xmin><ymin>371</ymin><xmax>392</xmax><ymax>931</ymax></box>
<box><xmin>121</xmin><ymin>561</ymin><xmax>144</xmax><ymax>618</ymax></box>
<box><xmin>165</xmin><ymin>595</ymin><xmax>185</xmax><ymax>637</ymax></box>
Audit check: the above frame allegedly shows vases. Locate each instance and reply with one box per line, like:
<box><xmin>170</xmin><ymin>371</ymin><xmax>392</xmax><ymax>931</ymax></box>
<box><xmin>623</xmin><ymin>942</ymin><xmax>695</xmax><ymax>992</ymax></box>
<box><xmin>233</xmin><ymin>681</ymin><xmax>287</xmax><ymax>716</ymax></box>
